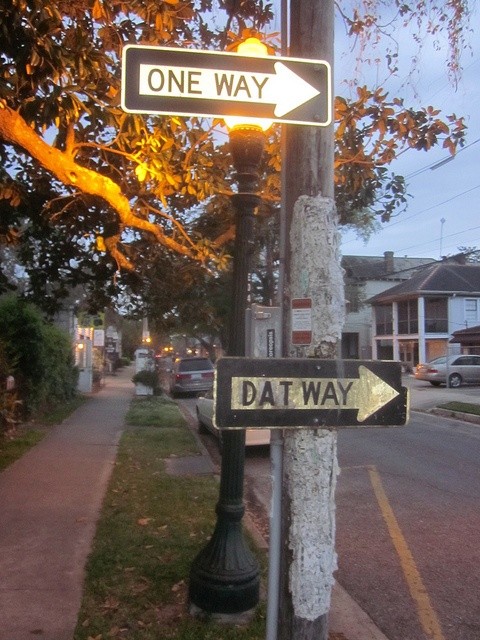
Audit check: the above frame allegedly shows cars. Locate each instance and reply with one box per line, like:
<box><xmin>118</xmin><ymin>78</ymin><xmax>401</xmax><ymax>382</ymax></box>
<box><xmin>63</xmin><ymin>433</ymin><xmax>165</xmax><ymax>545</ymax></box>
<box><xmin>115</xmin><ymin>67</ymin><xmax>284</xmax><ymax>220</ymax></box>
<box><xmin>169</xmin><ymin>356</ymin><xmax>214</xmax><ymax>397</ymax></box>
<box><xmin>195</xmin><ymin>386</ymin><xmax>271</xmax><ymax>457</ymax></box>
<box><xmin>415</xmin><ymin>354</ymin><xmax>480</xmax><ymax>387</ymax></box>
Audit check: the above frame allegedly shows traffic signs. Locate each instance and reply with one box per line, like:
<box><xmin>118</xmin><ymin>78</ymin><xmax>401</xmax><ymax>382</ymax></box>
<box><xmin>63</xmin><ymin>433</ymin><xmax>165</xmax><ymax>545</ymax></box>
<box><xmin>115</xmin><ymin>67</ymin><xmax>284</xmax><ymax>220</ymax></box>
<box><xmin>213</xmin><ymin>357</ymin><xmax>410</xmax><ymax>427</ymax></box>
<box><xmin>120</xmin><ymin>43</ymin><xmax>330</xmax><ymax>127</ymax></box>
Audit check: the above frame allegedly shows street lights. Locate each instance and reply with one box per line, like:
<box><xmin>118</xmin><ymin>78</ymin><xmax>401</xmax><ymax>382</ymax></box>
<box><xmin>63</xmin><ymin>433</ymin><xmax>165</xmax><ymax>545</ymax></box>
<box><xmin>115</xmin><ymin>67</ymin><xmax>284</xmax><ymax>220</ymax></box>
<box><xmin>187</xmin><ymin>35</ymin><xmax>277</xmax><ymax>615</ymax></box>
